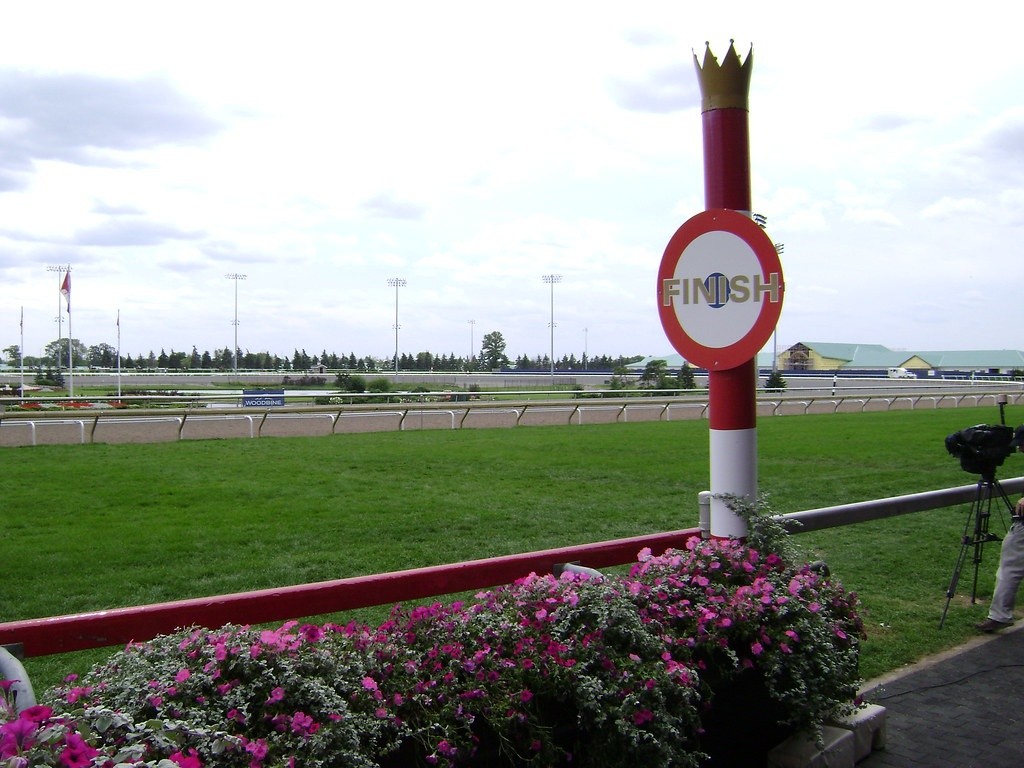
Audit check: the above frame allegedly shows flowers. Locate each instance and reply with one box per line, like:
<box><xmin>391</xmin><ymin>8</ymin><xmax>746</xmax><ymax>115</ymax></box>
<box><xmin>0</xmin><ymin>489</ymin><xmax>891</xmax><ymax>768</ymax></box>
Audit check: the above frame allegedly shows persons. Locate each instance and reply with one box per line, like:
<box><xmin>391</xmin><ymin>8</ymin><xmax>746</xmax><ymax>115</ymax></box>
<box><xmin>974</xmin><ymin>497</ymin><xmax>1024</xmax><ymax>631</ymax></box>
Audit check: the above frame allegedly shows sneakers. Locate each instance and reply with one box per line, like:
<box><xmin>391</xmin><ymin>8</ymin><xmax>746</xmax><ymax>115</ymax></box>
<box><xmin>975</xmin><ymin>618</ymin><xmax>1014</xmax><ymax>633</ymax></box>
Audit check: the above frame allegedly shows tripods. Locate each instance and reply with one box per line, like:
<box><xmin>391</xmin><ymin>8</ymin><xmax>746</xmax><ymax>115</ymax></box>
<box><xmin>938</xmin><ymin>466</ymin><xmax>1016</xmax><ymax>631</ymax></box>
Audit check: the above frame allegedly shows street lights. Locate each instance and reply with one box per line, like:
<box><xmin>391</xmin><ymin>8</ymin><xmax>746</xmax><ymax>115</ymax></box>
<box><xmin>466</xmin><ymin>320</ymin><xmax>477</xmax><ymax>363</ymax></box>
<box><xmin>224</xmin><ymin>272</ymin><xmax>247</xmax><ymax>386</ymax></box>
<box><xmin>386</xmin><ymin>277</ymin><xmax>407</xmax><ymax>375</ymax></box>
<box><xmin>46</xmin><ymin>265</ymin><xmax>73</xmax><ymax>382</ymax></box>
<box><xmin>542</xmin><ymin>274</ymin><xmax>564</xmax><ymax>389</ymax></box>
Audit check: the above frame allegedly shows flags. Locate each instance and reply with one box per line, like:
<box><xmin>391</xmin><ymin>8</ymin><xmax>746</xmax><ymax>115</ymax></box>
<box><xmin>61</xmin><ymin>272</ymin><xmax>71</xmax><ymax>313</ymax></box>
